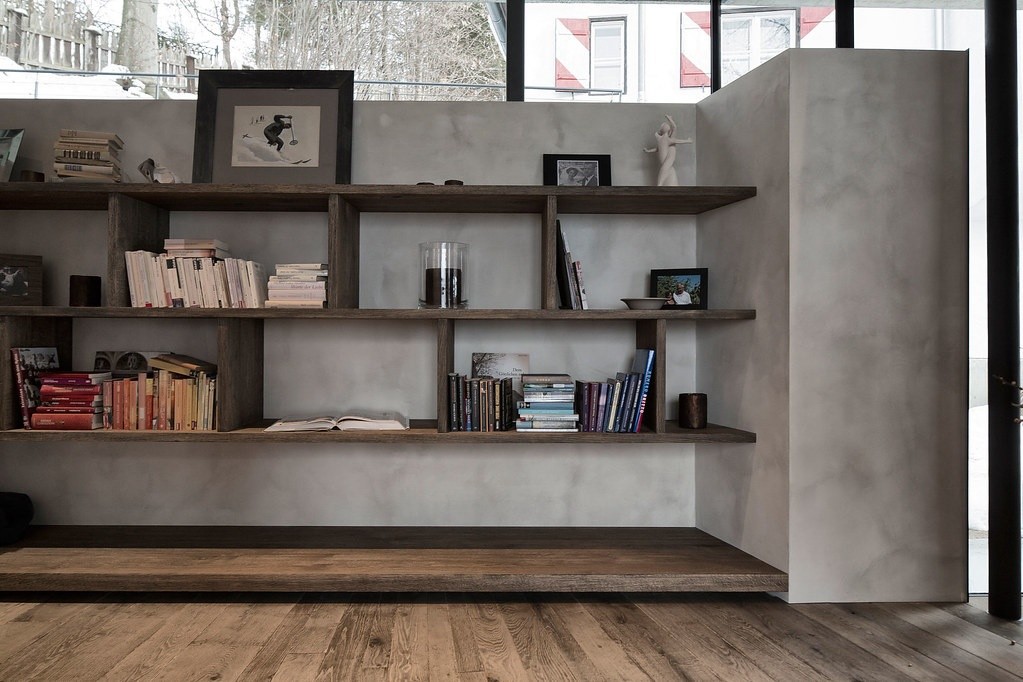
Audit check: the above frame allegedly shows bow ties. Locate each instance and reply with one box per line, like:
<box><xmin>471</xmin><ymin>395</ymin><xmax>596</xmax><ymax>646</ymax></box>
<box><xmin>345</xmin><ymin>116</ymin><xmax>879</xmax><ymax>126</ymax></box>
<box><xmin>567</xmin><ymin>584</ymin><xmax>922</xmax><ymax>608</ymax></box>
<box><xmin>585</xmin><ymin>177</ymin><xmax>588</xmax><ymax>181</ymax></box>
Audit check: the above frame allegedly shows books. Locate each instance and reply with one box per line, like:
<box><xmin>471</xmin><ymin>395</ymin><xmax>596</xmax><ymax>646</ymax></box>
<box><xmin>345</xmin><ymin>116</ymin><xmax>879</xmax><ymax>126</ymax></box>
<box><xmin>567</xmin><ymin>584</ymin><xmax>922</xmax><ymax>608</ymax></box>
<box><xmin>446</xmin><ymin>341</ymin><xmax>658</xmax><ymax>431</ymax></box>
<box><xmin>124</xmin><ymin>235</ymin><xmax>329</xmax><ymax>307</ymax></box>
<box><xmin>556</xmin><ymin>219</ymin><xmax>590</xmax><ymax>310</ymax></box>
<box><xmin>52</xmin><ymin>128</ymin><xmax>126</xmax><ymax>182</ymax></box>
<box><xmin>261</xmin><ymin>408</ymin><xmax>410</xmax><ymax>432</ymax></box>
<box><xmin>11</xmin><ymin>338</ymin><xmax>221</xmax><ymax>430</ymax></box>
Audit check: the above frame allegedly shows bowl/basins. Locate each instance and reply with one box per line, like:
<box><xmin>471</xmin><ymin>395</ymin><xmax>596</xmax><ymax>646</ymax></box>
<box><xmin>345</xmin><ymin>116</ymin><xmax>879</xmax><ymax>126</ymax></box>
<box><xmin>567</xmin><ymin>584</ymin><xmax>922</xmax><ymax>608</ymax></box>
<box><xmin>620</xmin><ymin>299</ymin><xmax>671</xmax><ymax>309</ymax></box>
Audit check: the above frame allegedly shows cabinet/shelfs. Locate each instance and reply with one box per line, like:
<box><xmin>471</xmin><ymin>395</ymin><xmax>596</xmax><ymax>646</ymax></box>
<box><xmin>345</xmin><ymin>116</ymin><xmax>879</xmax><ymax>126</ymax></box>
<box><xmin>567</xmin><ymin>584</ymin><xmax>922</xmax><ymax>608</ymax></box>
<box><xmin>0</xmin><ymin>182</ymin><xmax>788</xmax><ymax>592</ymax></box>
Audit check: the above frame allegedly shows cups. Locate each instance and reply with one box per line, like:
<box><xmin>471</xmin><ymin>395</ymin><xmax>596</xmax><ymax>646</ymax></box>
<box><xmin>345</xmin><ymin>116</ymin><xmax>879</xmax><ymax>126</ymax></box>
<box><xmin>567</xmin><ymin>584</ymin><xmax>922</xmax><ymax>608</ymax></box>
<box><xmin>419</xmin><ymin>242</ymin><xmax>467</xmax><ymax>310</ymax></box>
<box><xmin>679</xmin><ymin>393</ymin><xmax>708</xmax><ymax>428</ymax></box>
<box><xmin>69</xmin><ymin>275</ymin><xmax>102</xmax><ymax>307</ymax></box>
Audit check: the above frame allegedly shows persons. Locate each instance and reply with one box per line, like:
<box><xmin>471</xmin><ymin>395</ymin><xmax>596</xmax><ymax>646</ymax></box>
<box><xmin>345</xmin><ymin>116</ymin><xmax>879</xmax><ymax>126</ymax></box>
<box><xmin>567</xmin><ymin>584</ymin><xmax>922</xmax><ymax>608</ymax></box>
<box><xmin>642</xmin><ymin>114</ymin><xmax>678</xmax><ymax>187</ymax></box>
<box><xmin>654</xmin><ymin>122</ymin><xmax>693</xmax><ymax>187</ymax></box>
<box><xmin>664</xmin><ymin>283</ymin><xmax>693</xmax><ymax>306</ymax></box>
<box><xmin>557</xmin><ymin>166</ymin><xmax>583</xmax><ymax>187</ymax></box>
<box><xmin>580</xmin><ymin>162</ymin><xmax>597</xmax><ymax>187</ymax></box>
<box><xmin>19</xmin><ymin>350</ymin><xmax>59</xmax><ymax>406</ymax></box>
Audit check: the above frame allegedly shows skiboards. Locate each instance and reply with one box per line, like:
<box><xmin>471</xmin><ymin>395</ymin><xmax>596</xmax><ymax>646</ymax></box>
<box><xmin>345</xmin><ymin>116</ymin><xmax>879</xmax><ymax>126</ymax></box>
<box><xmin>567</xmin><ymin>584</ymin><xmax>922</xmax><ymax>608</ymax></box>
<box><xmin>244</xmin><ymin>133</ymin><xmax>312</xmax><ymax>164</ymax></box>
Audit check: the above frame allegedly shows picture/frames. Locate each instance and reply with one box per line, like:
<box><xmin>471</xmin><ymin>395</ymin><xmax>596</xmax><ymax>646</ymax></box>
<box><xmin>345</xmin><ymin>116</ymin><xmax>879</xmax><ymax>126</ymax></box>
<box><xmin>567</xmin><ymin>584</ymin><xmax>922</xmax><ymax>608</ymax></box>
<box><xmin>0</xmin><ymin>128</ymin><xmax>25</xmax><ymax>182</ymax></box>
<box><xmin>543</xmin><ymin>153</ymin><xmax>612</xmax><ymax>186</ymax></box>
<box><xmin>192</xmin><ymin>69</ymin><xmax>354</xmax><ymax>185</ymax></box>
<box><xmin>651</xmin><ymin>268</ymin><xmax>709</xmax><ymax>310</ymax></box>
<box><xmin>0</xmin><ymin>253</ymin><xmax>43</xmax><ymax>307</ymax></box>
